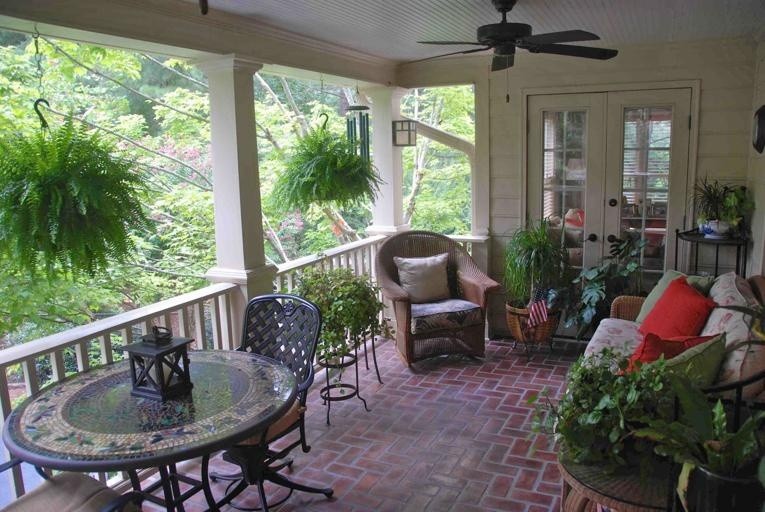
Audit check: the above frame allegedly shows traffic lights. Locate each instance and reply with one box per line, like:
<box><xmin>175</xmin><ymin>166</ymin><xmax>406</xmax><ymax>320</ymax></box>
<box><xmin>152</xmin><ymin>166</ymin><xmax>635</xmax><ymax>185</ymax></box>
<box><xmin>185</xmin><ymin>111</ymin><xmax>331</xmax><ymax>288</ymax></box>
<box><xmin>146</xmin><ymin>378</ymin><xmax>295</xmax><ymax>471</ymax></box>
<box><xmin>617</xmin><ymin>268</ymin><xmax>765</xmax><ymax>399</ymax></box>
<box><xmin>392</xmin><ymin>253</ymin><xmax>451</xmax><ymax>304</ymax></box>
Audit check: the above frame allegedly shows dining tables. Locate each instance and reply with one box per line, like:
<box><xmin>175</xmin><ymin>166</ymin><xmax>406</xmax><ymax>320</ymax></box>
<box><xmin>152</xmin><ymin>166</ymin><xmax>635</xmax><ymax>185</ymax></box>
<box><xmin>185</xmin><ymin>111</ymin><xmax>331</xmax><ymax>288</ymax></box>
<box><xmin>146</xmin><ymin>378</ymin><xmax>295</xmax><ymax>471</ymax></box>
<box><xmin>0</xmin><ymin>110</ymin><xmax>157</xmax><ymax>288</ymax></box>
<box><xmin>564</xmin><ymin>228</ymin><xmax>647</xmax><ymax>341</ymax></box>
<box><xmin>619</xmin><ymin>399</ymin><xmax>765</xmax><ymax>512</ymax></box>
<box><xmin>688</xmin><ymin>168</ymin><xmax>754</xmax><ymax>240</ymax></box>
<box><xmin>267</xmin><ymin>122</ymin><xmax>387</xmax><ymax>222</ymax></box>
<box><xmin>501</xmin><ymin>216</ymin><xmax>566</xmax><ymax>345</ymax></box>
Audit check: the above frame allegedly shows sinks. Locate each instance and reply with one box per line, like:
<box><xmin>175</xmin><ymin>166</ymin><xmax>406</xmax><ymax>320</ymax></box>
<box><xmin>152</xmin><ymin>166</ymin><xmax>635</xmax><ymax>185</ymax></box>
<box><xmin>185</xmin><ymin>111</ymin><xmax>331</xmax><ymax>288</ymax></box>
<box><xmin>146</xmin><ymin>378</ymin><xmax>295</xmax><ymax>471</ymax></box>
<box><xmin>552</xmin><ymin>275</ymin><xmax>765</xmax><ymax>436</ymax></box>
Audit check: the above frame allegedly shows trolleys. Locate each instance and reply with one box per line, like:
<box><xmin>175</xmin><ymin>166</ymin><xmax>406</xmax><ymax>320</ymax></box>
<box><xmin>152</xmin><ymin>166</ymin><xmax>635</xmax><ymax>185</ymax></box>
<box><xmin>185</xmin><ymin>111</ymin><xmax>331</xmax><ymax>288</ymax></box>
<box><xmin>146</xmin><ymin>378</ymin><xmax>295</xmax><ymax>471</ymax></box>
<box><xmin>392</xmin><ymin>0</ymin><xmax>619</xmax><ymax>103</ymax></box>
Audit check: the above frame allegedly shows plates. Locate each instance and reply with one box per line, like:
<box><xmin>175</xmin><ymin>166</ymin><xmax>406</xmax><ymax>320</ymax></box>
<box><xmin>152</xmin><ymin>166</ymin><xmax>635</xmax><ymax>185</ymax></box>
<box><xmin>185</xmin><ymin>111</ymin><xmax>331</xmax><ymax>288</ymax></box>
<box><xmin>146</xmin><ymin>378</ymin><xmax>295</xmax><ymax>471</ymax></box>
<box><xmin>317</xmin><ymin>317</ymin><xmax>383</xmax><ymax>425</ymax></box>
<box><xmin>620</xmin><ymin>220</ymin><xmax>666</xmax><ymax>258</ymax></box>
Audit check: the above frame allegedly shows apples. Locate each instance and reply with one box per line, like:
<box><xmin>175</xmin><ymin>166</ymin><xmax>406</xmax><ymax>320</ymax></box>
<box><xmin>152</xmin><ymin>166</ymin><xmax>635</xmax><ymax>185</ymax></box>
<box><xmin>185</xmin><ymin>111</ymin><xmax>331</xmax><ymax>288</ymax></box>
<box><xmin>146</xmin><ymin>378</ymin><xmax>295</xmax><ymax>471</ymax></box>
<box><xmin>145</xmin><ymin>361</ymin><xmax>172</xmax><ymax>383</ymax></box>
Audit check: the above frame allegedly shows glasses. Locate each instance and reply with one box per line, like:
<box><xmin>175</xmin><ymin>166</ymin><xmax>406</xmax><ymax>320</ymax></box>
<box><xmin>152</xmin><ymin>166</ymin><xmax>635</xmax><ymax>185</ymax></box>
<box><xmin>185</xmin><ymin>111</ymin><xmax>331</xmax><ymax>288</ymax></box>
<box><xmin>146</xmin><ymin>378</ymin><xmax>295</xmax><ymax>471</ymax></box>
<box><xmin>560</xmin><ymin>151</ymin><xmax>586</xmax><ymax>181</ymax></box>
<box><xmin>393</xmin><ymin>120</ymin><xmax>418</xmax><ymax>146</ymax></box>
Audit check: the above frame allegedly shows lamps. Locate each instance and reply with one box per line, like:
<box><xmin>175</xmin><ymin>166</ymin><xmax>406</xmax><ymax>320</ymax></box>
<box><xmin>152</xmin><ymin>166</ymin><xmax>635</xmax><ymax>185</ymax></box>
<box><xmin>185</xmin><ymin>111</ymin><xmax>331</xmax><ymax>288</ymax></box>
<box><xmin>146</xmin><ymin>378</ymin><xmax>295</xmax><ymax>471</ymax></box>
<box><xmin>0</xmin><ymin>453</ymin><xmax>144</xmax><ymax>512</ymax></box>
<box><xmin>202</xmin><ymin>294</ymin><xmax>334</xmax><ymax>511</ymax></box>
<box><xmin>374</xmin><ymin>230</ymin><xmax>501</xmax><ymax>367</ymax></box>
